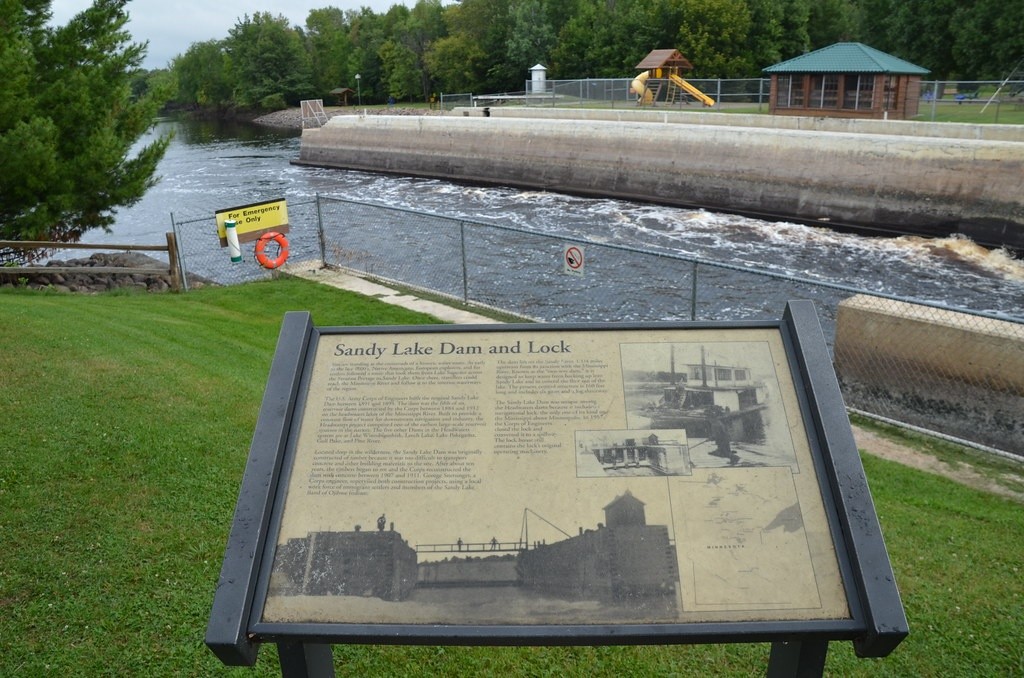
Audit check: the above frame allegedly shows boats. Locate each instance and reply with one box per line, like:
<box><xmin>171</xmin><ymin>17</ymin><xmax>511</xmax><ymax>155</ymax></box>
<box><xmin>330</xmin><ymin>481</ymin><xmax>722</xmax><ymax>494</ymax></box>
<box><xmin>644</xmin><ymin>342</ymin><xmax>730</xmax><ymax>441</ymax></box>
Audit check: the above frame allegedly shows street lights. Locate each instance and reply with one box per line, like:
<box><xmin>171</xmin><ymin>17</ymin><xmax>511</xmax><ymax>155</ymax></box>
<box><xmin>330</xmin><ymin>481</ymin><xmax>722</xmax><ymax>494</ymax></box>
<box><xmin>354</xmin><ymin>73</ymin><xmax>361</xmax><ymax>106</ymax></box>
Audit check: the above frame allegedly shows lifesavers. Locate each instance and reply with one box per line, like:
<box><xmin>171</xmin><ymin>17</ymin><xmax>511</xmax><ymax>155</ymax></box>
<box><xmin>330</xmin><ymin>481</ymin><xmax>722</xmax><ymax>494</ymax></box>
<box><xmin>256</xmin><ymin>231</ymin><xmax>288</xmax><ymax>269</ymax></box>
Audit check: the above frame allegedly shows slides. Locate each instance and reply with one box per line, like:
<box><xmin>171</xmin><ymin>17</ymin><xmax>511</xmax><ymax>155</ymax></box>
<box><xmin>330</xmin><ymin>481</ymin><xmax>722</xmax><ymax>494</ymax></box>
<box><xmin>670</xmin><ymin>74</ymin><xmax>715</xmax><ymax>107</ymax></box>
<box><xmin>631</xmin><ymin>70</ymin><xmax>653</xmax><ymax>105</ymax></box>
